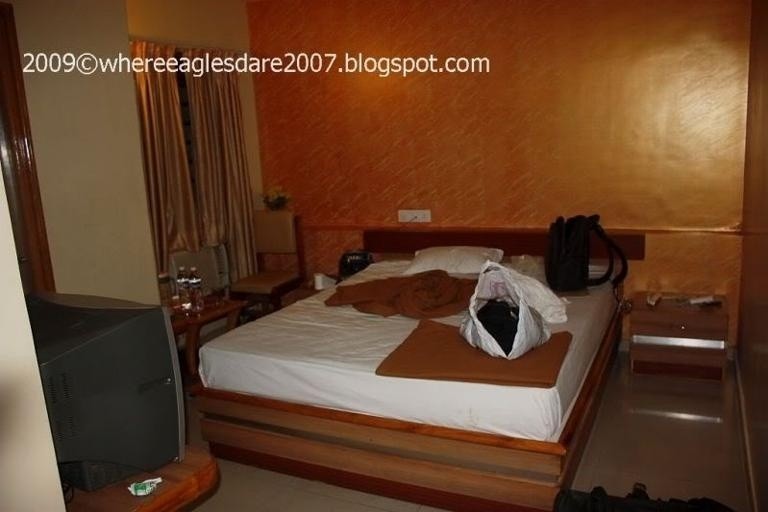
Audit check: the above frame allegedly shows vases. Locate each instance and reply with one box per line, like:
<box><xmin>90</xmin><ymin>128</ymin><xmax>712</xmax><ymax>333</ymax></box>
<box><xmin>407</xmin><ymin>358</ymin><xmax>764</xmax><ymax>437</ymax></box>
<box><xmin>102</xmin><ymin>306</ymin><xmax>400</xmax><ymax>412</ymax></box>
<box><xmin>267</xmin><ymin>199</ymin><xmax>286</xmax><ymax>210</ymax></box>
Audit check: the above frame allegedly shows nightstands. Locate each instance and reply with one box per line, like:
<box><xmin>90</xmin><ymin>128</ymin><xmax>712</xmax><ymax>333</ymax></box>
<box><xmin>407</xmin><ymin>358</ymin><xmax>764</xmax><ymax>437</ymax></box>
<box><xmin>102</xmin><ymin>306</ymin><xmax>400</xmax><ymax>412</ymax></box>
<box><xmin>629</xmin><ymin>290</ymin><xmax>730</xmax><ymax>381</ymax></box>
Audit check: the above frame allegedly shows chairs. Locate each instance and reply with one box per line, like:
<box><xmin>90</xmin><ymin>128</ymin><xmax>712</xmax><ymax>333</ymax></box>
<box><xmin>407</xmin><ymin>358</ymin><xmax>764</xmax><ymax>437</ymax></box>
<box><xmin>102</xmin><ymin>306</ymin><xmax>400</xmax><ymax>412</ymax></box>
<box><xmin>229</xmin><ymin>209</ymin><xmax>306</xmax><ymax>328</ymax></box>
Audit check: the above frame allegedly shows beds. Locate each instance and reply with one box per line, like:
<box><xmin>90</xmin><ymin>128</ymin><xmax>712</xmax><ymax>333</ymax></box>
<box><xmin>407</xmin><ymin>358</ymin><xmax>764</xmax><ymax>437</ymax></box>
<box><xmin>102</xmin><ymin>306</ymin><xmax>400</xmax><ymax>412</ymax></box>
<box><xmin>171</xmin><ymin>295</ymin><xmax>249</xmax><ymax>375</ymax></box>
<box><xmin>189</xmin><ymin>226</ymin><xmax>645</xmax><ymax>512</ymax></box>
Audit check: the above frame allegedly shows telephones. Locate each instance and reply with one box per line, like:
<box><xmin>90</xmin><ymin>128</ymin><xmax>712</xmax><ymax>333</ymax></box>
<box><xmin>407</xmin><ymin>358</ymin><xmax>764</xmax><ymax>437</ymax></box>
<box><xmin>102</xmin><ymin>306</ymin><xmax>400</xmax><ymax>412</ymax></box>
<box><xmin>340</xmin><ymin>253</ymin><xmax>369</xmax><ymax>276</ymax></box>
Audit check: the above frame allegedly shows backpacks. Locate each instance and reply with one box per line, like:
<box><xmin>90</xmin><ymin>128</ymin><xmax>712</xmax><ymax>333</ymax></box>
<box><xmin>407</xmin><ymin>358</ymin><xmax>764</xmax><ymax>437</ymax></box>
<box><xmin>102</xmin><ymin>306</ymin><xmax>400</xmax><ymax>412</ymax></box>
<box><xmin>544</xmin><ymin>213</ymin><xmax>628</xmax><ymax>292</ymax></box>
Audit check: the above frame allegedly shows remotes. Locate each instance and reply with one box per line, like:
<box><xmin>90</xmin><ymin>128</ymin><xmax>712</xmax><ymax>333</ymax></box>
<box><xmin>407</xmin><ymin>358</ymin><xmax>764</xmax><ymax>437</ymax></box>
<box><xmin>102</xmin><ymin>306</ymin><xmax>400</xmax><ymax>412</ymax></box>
<box><xmin>699</xmin><ymin>301</ymin><xmax>722</xmax><ymax>309</ymax></box>
<box><xmin>690</xmin><ymin>294</ymin><xmax>715</xmax><ymax>305</ymax></box>
<box><xmin>650</xmin><ymin>292</ymin><xmax>662</xmax><ymax>306</ymax></box>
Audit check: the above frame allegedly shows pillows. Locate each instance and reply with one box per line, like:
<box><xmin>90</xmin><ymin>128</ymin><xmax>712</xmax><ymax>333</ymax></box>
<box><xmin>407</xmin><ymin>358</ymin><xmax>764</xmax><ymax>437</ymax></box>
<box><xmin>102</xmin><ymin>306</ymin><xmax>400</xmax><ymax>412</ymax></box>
<box><xmin>400</xmin><ymin>245</ymin><xmax>504</xmax><ymax>275</ymax></box>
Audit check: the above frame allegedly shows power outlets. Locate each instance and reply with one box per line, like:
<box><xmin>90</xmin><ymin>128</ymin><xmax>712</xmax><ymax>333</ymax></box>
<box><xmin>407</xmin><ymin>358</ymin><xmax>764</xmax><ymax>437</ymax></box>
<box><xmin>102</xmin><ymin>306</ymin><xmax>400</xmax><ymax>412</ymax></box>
<box><xmin>398</xmin><ymin>209</ymin><xmax>431</xmax><ymax>223</ymax></box>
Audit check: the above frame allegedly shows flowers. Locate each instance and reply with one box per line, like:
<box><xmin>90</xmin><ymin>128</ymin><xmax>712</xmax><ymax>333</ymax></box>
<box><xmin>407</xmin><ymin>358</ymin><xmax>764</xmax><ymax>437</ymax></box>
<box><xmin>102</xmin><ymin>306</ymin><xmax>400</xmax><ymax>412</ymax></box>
<box><xmin>259</xmin><ymin>179</ymin><xmax>292</xmax><ymax>201</ymax></box>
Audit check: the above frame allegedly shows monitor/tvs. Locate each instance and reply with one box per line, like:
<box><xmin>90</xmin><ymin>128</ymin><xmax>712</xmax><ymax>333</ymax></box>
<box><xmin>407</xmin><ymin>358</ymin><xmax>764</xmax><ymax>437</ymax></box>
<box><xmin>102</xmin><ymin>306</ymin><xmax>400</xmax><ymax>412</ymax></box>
<box><xmin>24</xmin><ymin>293</ymin><xmax>188</xmax><ymax>493</ymax></box>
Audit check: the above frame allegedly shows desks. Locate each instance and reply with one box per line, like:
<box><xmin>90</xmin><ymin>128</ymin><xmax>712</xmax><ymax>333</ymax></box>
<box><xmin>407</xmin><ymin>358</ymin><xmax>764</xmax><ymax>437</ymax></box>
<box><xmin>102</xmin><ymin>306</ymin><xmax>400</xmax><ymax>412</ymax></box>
<box><xmin>61</xmin><ymin>439</ymin><xmax>221</xmax><ymax>512</ymax></box>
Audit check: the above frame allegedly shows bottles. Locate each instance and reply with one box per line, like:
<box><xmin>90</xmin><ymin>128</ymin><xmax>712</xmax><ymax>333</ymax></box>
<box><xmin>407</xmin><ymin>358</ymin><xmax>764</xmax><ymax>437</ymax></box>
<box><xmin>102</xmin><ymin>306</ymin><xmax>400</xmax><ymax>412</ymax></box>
<box><xmin>176</xmin><ymin>267</ymin><xmax>191</xmax><ymax>312</ymax></box>
<box><xmin>189</xmin><ymin>267</ymin><xmax>204</xmax><ymax>313</ymax></box>
